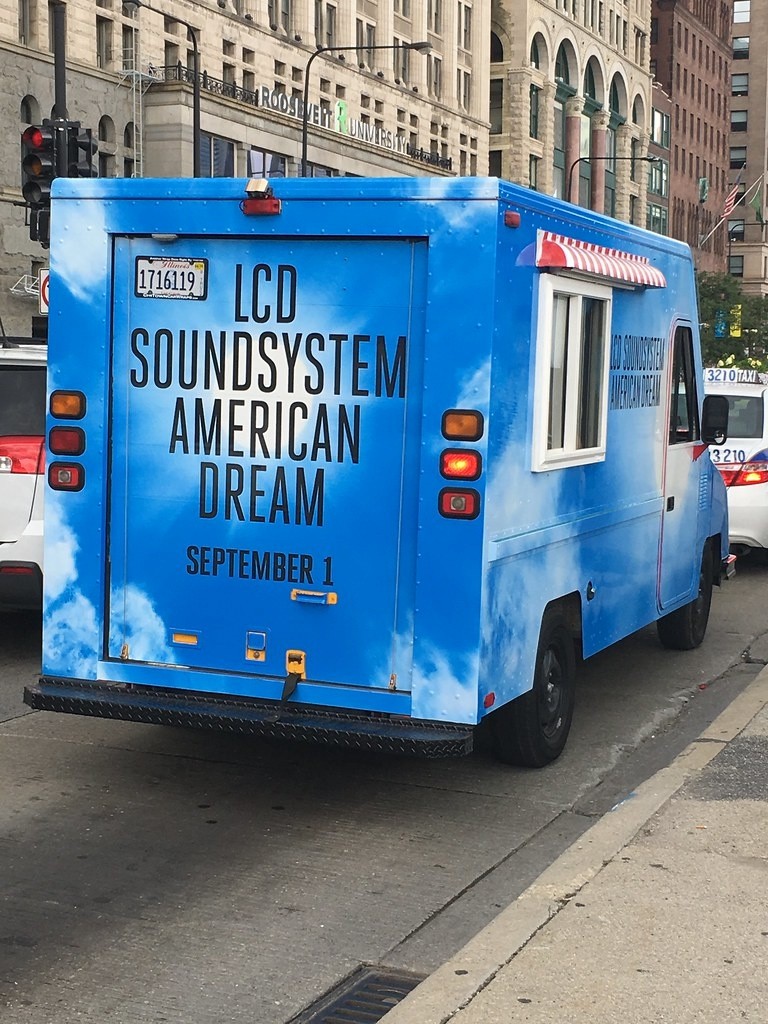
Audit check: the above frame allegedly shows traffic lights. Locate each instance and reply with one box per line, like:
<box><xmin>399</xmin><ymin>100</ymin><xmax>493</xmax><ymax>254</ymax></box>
<box><xmin>24</xmin><ymin>205</ymin><xmax>50</xmax><ymax>249</ymax></box>
<box><xmin>20</xmin><ymin>124</ymin><xmax>54</xmax><ymax>207</ymax></box>
<box><xmin>67</xmin><ymin>121</ymin><xmax>101</xmax><ymax>179</ymax></box>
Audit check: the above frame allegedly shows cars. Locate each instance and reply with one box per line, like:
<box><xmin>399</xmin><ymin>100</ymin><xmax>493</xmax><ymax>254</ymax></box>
<box><xmin>0</xmin><ymin>340</ymin><xmax>47</xmax><ymax>624</ymax></box>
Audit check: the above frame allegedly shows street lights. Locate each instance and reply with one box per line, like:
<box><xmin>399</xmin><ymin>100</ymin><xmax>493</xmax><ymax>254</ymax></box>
<box><xmin>124</xmin><ymin>0</ymin><xmax>203</xmax><ymax>180</ymax></box>
<box><xmin>300</xmin><ymin>41</ymin><xmax>432</xmax><ymax>179</ymax></box>
<box><xmin>567</xmin><ymin>157</ymin><xmax>661</xmax><ymax>207</ymax></box>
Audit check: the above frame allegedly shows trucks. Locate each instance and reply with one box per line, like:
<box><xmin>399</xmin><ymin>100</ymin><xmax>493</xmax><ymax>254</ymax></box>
<box><xmin>19</xmin><ymin>176</ymin><xmax>736</xmax><ymax>770</ymax></box>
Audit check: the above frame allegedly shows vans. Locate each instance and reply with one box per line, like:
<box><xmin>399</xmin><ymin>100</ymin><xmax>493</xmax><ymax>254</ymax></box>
<box><xmin>700</xmin><ymin>367</ymin><xmax>768</xmax><ymax>558</ymax></box>
